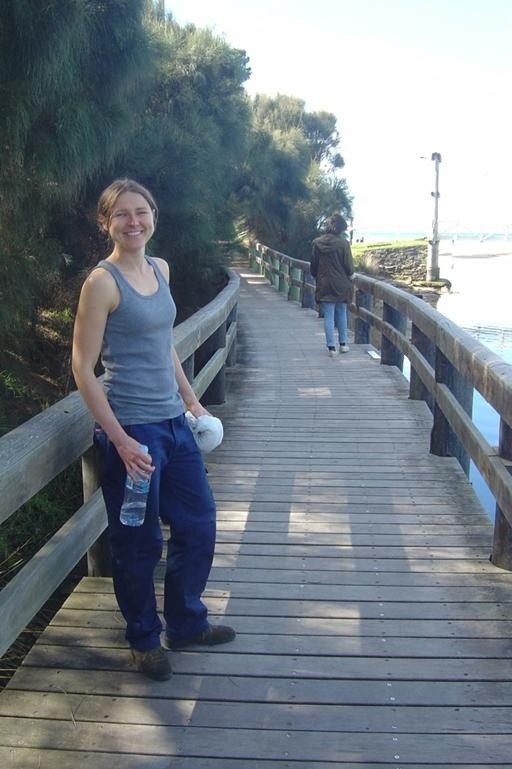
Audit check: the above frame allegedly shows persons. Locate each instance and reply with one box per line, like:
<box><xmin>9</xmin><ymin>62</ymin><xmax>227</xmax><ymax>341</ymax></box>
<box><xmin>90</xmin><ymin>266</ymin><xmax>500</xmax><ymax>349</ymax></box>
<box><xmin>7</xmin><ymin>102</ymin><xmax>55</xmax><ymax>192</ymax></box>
<box><xmin>69</xmin><ymin>178</ymin><xmax>236</xmax><ymax>681</ymax></box>
<box><xmin>310</xmin><ymin>215</ymin><xmax>356</xmax><ymax>359</ymax></box>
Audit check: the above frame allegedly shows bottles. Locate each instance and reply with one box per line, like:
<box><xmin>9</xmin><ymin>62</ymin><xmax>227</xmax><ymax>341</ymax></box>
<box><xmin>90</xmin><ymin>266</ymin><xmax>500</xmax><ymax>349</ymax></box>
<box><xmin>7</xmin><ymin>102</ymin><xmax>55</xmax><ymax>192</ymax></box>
<box><xmin>117</xmin><ymin>446</ymin><xmax>150</xmax><ymax>529</ymax></box>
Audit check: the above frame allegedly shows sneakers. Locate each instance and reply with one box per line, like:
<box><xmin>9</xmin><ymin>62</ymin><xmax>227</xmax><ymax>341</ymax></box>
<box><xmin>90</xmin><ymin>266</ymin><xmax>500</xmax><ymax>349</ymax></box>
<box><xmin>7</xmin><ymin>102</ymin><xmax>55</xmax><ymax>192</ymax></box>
<box><xmin>327</xmin><ymin>349</ymin><xmax>338</xmax><ymax>357</ymax></box>
<box><xmin>339</xmin><ymin>345</ymin><xmax>350</xmax><ymax>353</ymax></box>
<box><xmin>164</xmin><ymin>625</ymin><xmax>236</xmax><ymax>648</ymax></box>
<box><xmin>131</xmin><ymin>645</ymin><xmax>172</xmax><ymax>681</ymax></box>
